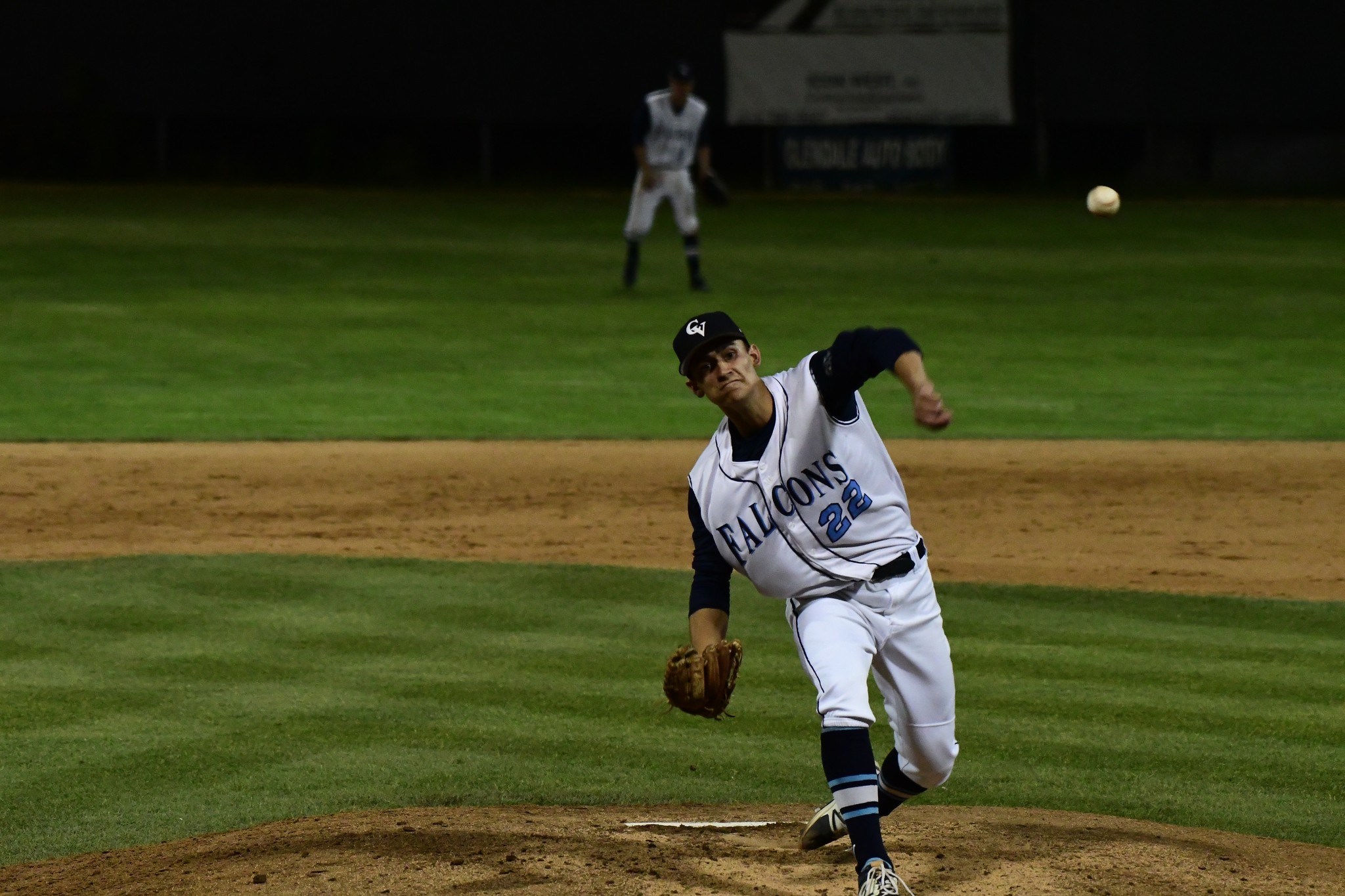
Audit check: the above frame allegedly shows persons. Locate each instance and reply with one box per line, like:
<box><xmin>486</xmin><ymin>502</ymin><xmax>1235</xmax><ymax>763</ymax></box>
<box><xmin>663</xmin><ymin>313</ymin><xmax>959</xmax><ymax>896</ymax></box>
<box><xmin>619</xmin><ymin>62</ymin><xmax>716</xmax><ymax>292</ymax></box>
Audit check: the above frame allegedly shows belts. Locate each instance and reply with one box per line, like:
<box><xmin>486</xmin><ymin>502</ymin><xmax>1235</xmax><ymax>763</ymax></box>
<box><xmin>854</xmin><ymin>538</ymin><xmax>926</xmax><ymax>583</ymax></box>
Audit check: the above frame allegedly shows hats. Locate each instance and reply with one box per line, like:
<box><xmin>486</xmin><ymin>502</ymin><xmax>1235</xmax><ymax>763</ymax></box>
<box><xmin>673</xmin><ymin>310</ymin><xmax>742</xmax><ymax>376</ymax></box>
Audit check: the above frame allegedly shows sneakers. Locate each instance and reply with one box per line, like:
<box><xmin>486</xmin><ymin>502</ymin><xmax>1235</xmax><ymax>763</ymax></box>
<box><xmin>854</xmin><ymin>855</ymin><xmax>914</xmax><ymax>896</ymax></box>
<box><xmin>800</xmin><ymin>762</ymin><xmax>881</xmax><ymax>850</ymax></box>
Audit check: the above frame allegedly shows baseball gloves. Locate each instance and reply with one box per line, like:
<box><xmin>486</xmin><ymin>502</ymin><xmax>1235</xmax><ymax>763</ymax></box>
<box><xmin>663</xmin><ymin>638</ymin><xmax>743</xmax><ymax>719</ymax></box>
<box><xmin>701</xmin><ymin>174</ymin><xmax>728</xmax><ymax>208</ymax></box>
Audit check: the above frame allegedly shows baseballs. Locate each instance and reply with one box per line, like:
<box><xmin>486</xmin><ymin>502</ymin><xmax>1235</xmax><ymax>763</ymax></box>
<box><xmin>1085</xmin><ymin>185</ymin><xmax>1119</xmax><ymax>215</ymax></box>
